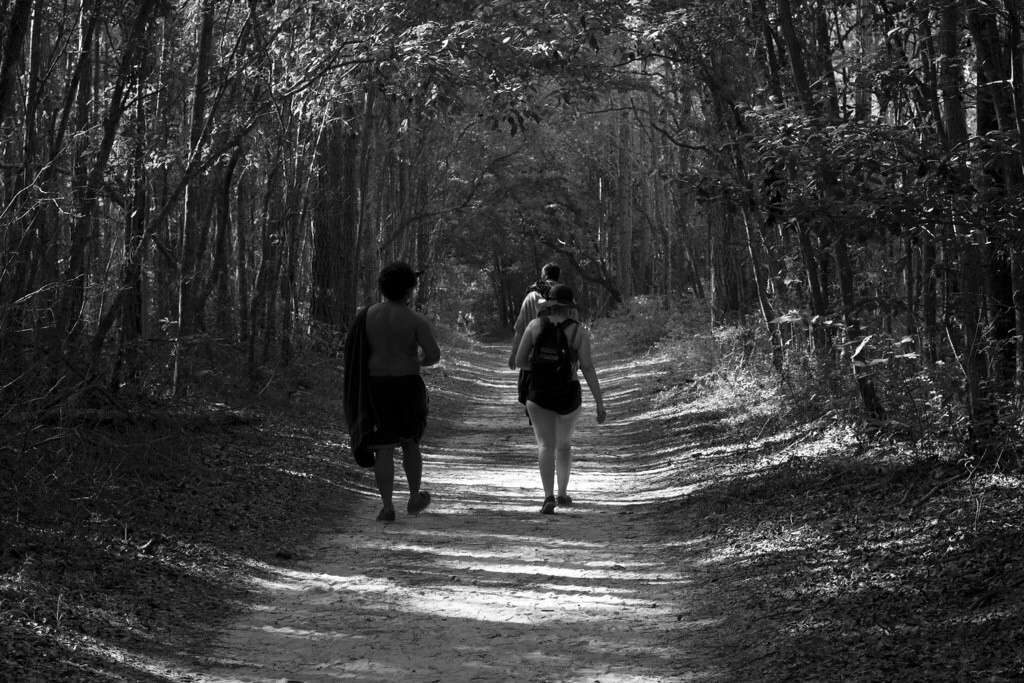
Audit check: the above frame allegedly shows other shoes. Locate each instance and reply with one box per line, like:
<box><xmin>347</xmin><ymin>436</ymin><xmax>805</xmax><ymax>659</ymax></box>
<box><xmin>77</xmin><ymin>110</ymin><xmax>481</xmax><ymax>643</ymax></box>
<box><xmin>556</xmin><ymin>495</ymin><xmax>574</xmax><ymax>504</ymax></box>
<box><xmin>407</xmin><ymin>491</ymin><xmax>430</xmax><ymax>514</ymax></box>
<box><xmin>377</xmin><ymin>508</ymin><xmax>395</xmax><ymax>520</ymax></box>
<box><xmin>540</xmin><ymin>496</ymin><xmax>557</xmax><ymax>514</ymax></box>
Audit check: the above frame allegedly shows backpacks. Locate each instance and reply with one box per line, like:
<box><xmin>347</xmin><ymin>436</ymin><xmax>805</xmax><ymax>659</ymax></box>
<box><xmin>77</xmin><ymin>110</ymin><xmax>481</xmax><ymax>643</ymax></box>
<box><xmin>530</xmin><ymin>316</ymin><xmax>580</xmax><ymax>393</ymax></box>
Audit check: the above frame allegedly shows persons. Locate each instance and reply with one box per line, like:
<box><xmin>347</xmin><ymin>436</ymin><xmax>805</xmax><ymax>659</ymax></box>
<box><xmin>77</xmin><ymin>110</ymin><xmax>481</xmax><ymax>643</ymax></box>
<box><xmin>354</xmin><ymin>260</ymin><xmax>441</xmax><ymax>522</ymax></box>
<box><xmin>455</xmin><ymin>309</ymin><xmax>478</xmax><ymax>338</ymax></box>
<box><xmin>509</xmin><ymin>264</ymin><xmax>579</xmax><ymax>426</ymax></box>
<box><xmin>516</xmin><ymin>285</ymin><xmax>606</xmax><ymax>513</ymax></box>
